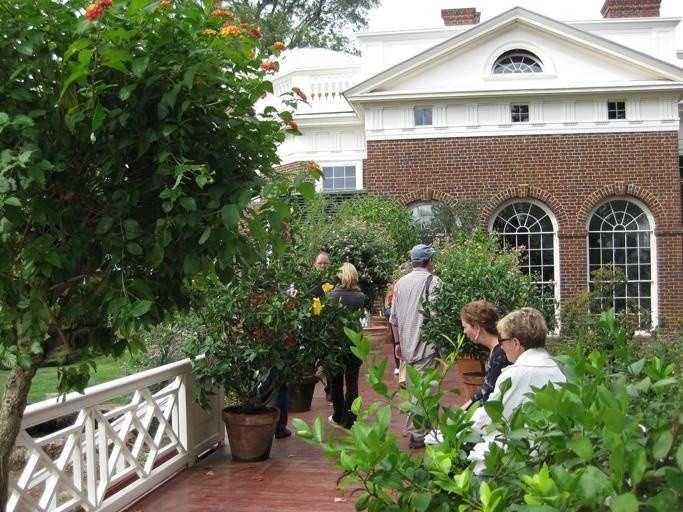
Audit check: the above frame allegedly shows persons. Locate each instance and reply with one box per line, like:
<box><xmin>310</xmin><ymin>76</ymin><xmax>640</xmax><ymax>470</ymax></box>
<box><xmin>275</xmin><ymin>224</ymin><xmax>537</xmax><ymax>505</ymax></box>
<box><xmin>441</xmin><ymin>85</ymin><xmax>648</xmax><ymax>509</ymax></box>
<box><xmin>455</xmin><ymin>306</ymin><xmax>569</xmax><ymax>510</ymax></box>
<box><xmin>388</xmin><ymin>242</ymin><xmax>447</xmax><ymax>448</ymax></box>
<box><xmin>383</xmin><ymin>278</ymin><xmax>399</xmax><ymax>377</ymax></box>
<box><xmin>302</xmin><ymin>261</ymin><xmax>367</xmax><ymax>432</ymax></box>
<box><xmin>306</xmin><ymin>251</ymin><xmax>334</xmax><ymax>403</ymax></box>
<box><xmin>416</xmin><ymin>299</ymin><xmax>511</xmax><ymax>484</ymax></box>
<box><xmin>243</xmin><ymin>266</ymin><xmax>298</xmax><ymax>440</ymax></box>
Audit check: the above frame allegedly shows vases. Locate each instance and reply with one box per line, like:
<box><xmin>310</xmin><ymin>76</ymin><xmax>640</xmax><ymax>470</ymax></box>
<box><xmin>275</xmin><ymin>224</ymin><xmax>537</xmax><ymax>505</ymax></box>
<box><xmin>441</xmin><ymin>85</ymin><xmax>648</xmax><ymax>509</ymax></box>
<box><xmin>459</xmin><ymin>372</ymin><xmax>484</xmax><ymax>402</ymax></box>
<box><xmin>284</xmin><ymin>379</ymin><xmax>318</xmax><ymax>412</ymax></box>
<box><xmin>221</xmin><ymin>406</ymin><xmax>279</xmax><ymax>462</ymax></box>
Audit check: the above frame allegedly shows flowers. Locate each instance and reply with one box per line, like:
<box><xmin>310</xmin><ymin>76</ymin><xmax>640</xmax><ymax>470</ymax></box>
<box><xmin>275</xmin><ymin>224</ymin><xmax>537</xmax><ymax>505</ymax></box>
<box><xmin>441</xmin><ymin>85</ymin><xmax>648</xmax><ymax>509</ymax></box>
<box><xmin>418</xmin><ymin>225</ymin><xmax>559</xmax><ymax>373</ymax></box>
<box><xmin>317</xmin><ymin>216</ymin><xmax>399</xmax><ymax>314</ymax></box>
<box><xmin>273</xmin><ymin>281</ymin><xmax>368</xmax><ymax>387</ymax></box>
<box><xmin>183</xmin><ymin>272</ymin><xmax>311</xmax><ymax>410</ymax></box>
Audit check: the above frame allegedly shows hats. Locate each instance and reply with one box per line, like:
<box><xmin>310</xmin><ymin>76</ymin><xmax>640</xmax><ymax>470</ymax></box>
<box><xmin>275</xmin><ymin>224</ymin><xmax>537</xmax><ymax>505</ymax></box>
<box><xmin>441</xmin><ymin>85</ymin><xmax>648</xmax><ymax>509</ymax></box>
<box><xmin>409</xmin><ymin>242</ymin><xmax>436</xmax><ymax>263</ymax></box>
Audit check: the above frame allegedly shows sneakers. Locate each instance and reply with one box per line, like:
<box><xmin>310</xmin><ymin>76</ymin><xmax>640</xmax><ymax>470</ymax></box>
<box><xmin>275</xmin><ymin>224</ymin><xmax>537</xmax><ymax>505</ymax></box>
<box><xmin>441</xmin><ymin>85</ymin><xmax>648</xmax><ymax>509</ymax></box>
<box><xmin>273</xmin><ymin>427</ymin><xmax>292</xmax><ymax>439</ymax></box>
<box><xmin>408</xmin><ymin>433</ymin><xmax>427</xmax><ymax>449</ymax></box>
<box><xmin>326</xmin><ymin>413</ymin><xmax>344</xmax><ymax>426</ymax></box>
<box><xmin>392</xmin><ymin>366</ymin><xmax>400</xmax><ymax>375</ymax></box>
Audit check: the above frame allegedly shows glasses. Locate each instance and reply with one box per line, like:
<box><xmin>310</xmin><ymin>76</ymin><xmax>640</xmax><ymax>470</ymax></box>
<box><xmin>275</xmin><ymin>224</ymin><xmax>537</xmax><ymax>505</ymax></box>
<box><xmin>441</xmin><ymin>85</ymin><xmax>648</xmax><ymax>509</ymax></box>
<box><xmin>496</xmin><ymin>335</ymin><xmax>513</xmax><ymax>345</ymax></box>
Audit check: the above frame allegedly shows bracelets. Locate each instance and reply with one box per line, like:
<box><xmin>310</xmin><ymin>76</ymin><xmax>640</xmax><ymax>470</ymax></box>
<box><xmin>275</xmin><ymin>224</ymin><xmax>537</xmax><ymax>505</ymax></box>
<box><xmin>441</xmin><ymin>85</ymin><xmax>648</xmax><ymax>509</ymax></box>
<box><xmin>393</xmin><ymin>342</ymin><xmax>399</xmax><ymax>345</ymax></box>
